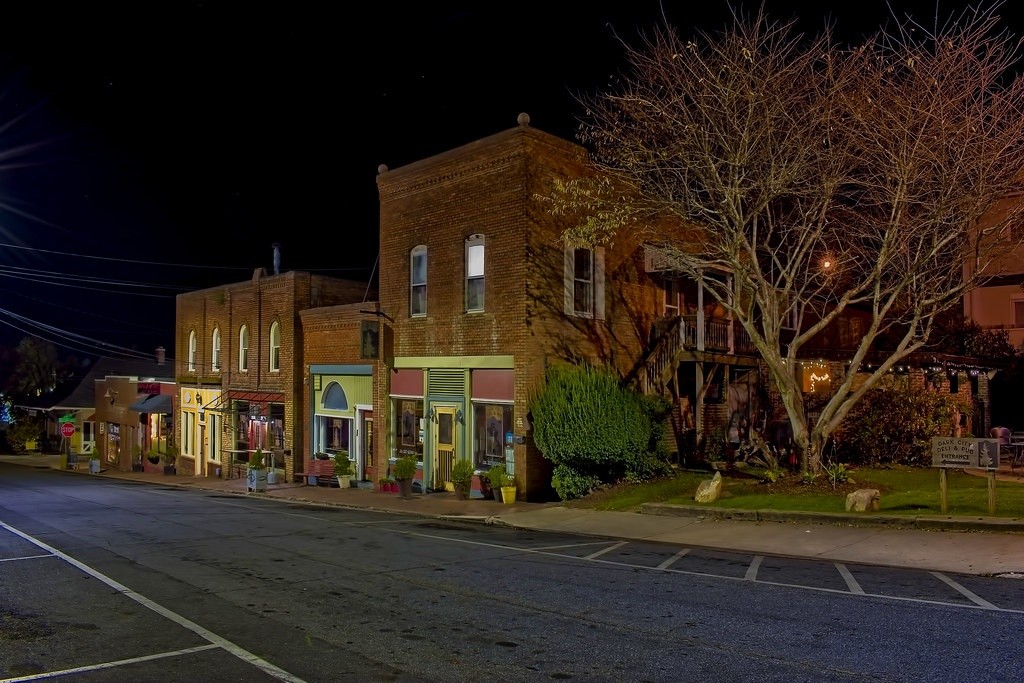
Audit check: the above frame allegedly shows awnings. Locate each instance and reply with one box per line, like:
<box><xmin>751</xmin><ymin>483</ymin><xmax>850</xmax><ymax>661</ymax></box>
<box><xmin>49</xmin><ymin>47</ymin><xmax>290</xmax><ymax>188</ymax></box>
<box><xmin>130</xmin><ymin>393</ymin><xmax>172</xmax><ymax>414</ymax></box>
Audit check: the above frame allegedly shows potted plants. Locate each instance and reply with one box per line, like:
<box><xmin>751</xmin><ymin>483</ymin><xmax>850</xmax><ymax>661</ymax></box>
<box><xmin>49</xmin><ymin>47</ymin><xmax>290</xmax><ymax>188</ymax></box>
<box><xmin>247</xmin><ymin>449</ymin><xmax>268</xmax><ymax>493</ymax></box>
<box><xmin>394</xmin><ymin>454</ymin><xmax>418</xmax><ymax>497</ymax></box>
<box><xmin>164</xmin><ymin>446</ymin><xmax>178</xmax><ymax>475</ymax></box>
<box><xmin>481</xmin><ymin>463</ymin><xmax>506</xmax><ymax>503</ymax></box>
<box><xmin>451</xmin><ymin>460</ymin><xmax>475</xmax><ymax>500</ymax></box>
<box><xmin>89</xmin><ymin>448</ymin><xmax>100</xmax><ymax>474</ymax></box>
<box><xmin>131</xmin><ymin>445</ymin><xmax>142</xmax><ymax>472</ymax></box>
<box><xmin>500</xmin><ymin>473</ymin><xmax>516</xmax><ymax>504</ymax></box>
<box><xmin>147</xmin><ymin>450</ymin><xmax>159</xmax><ymax>465</ymax></box>
<box><xmin>379</xmin><ymin>477</ymin><xmax>398</xmax><ymax>494</ymax></box>
<box><xmin>334</xmin><ymin>450</ymin><xmax>354</xmax><ymax>488</ymax></box>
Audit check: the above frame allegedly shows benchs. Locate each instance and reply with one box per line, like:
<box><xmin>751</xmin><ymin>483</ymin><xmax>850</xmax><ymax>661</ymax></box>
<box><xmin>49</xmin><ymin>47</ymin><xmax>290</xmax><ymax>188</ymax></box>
<box><xmin>294</xmin><ymin>460</ymin><xmax>335</xmax><ymax>488</ymax></box>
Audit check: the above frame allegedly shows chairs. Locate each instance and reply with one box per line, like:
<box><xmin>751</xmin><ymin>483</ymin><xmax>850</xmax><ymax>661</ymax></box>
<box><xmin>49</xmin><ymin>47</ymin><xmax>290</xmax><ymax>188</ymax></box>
<box><xmin>989</xmin><ymin>427</ymin><xmax>1014</xmax><ymax>465</ymax></box>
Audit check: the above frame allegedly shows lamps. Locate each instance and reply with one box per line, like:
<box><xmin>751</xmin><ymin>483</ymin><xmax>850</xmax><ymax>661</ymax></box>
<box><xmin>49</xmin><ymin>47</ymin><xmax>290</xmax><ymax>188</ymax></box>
<box><xmin>425</xmin><ymin>409</ymin><xmax>433</xmax><ymax>419</ymax></box>
<box><xmin>195</xmin><ymin>392</ymin><xmax>201</xmax><ymax>404</ymax></box>
<box><xmin>454</xmin><ymin>410</ymin><xmax>463</xmax><ymax>422</ymax></box>
<box><xmin>104</xmin><ymin>387</ymin><xmax>119</xmax><ymax>398</ymax></box>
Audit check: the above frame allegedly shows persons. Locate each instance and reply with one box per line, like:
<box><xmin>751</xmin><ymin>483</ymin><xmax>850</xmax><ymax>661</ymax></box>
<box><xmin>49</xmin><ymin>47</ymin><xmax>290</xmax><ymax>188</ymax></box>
<box><xmin>488</xmin><ymin>423</ymin><xmax>502</xmax><ymax>455</ymax></box>
<box><xmin>404</xmin><ymin>411</ymin><xmax>412</xmax><ymax>436</ymax></box>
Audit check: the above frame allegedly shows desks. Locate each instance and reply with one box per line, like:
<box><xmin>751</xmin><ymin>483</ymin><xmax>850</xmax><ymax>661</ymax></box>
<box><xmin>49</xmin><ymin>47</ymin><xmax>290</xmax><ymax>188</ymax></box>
<box><xmin>246</xmin><ymin>450</ymin><xmax>275</xmax><ymax>473</ymax></box>
<box><xmin>1000</xmin><ymin>435</ymin><xmax>1024</xmax><ymax>466</ymax></box>
<box><xmin>219</xmin><ymin>449</ymin><xmax>246</xmax><ymax>480</ymax></box>
